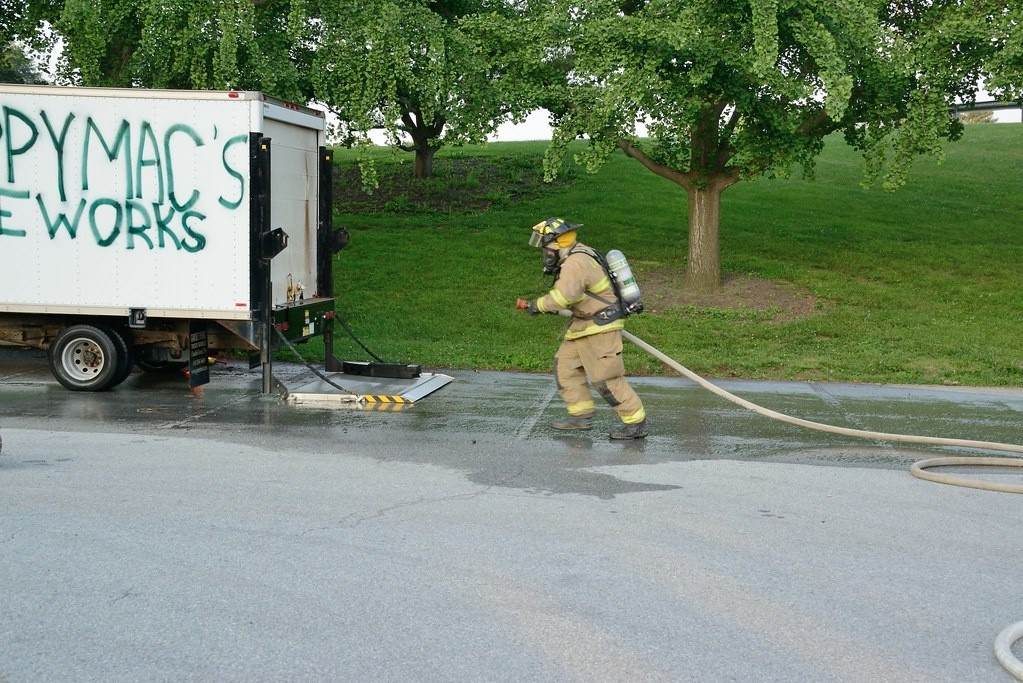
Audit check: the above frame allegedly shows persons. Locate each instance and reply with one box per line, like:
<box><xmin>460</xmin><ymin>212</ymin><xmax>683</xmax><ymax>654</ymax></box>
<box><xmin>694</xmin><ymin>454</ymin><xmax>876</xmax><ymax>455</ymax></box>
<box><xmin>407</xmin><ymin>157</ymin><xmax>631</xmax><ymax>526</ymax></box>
<box><xmin>529</xmin><ymin>217</ymin><xmax>650</xmax><ymax>439</ymax></box>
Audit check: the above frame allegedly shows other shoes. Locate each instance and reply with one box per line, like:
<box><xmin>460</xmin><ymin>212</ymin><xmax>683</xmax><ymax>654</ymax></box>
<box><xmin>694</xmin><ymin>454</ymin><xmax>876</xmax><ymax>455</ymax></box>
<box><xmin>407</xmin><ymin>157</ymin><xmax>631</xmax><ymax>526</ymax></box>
<box><xmin>551</xmin><ymin>415</ymin><xmax>593</xmax><ymax>431</ymax></box>
<box><xmin>609</xmin><ymin>419</ymin><xmax>649</xmax><ymax>439</ymax></box>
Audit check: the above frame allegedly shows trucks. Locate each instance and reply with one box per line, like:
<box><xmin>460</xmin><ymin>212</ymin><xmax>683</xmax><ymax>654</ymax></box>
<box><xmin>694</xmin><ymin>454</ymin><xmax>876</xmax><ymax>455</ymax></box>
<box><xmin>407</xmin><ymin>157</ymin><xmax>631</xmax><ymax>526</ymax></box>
<box><xmin>0</xmin><ymin>84</ymin><xmax>352</xmax><ymax>398</ymax></box>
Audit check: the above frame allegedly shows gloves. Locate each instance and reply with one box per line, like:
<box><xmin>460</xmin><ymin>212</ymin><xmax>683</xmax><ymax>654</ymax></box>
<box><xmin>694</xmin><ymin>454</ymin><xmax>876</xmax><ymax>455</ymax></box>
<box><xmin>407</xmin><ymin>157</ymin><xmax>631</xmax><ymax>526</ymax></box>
<box><xmin>530</xmin><ymin>299</ymin><xmax>540</xmax><ymax>314</ymax></box>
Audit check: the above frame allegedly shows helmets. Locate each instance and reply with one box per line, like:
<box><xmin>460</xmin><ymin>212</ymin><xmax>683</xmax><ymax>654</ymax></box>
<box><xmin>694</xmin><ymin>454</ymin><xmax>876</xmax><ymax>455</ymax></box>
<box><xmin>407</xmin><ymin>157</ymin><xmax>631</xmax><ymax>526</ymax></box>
<box><xmin>528</xmin><ymin>216</ymin><xmax>585</xmax><ymax>248</ymax></box>
